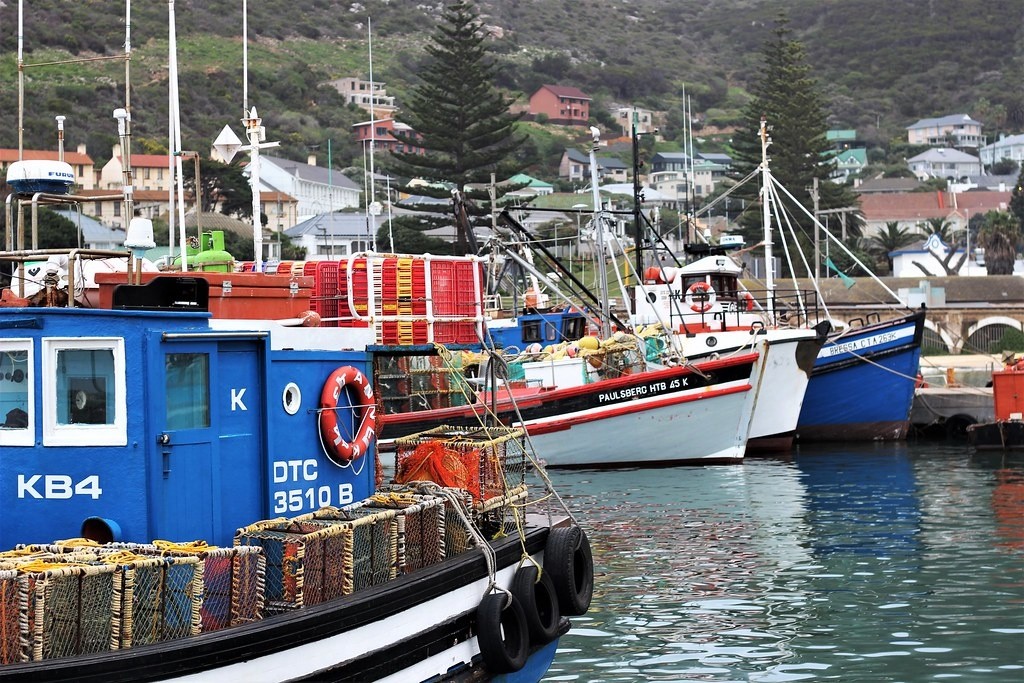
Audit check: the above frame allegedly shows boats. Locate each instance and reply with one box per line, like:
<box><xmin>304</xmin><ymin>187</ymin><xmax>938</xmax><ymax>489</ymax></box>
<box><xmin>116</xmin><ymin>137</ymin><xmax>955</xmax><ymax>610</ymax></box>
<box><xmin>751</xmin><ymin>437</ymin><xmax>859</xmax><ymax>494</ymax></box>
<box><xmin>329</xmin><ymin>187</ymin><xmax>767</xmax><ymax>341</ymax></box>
<box><xmin>2</xmin><ymin>0</ymin><xmax>1024</xmax><ymax>683</ymax></box>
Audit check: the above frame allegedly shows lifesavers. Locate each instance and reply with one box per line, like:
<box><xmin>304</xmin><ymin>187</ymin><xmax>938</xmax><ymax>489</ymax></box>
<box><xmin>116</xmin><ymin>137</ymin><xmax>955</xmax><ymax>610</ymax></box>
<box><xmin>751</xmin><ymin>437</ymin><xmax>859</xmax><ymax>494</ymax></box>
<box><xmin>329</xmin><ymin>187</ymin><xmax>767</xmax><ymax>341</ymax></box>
<box><xmin>512</xmin><ymin>565</ymin><xmax>560</xmax><ymax>644</ymax></box>
<box><xmin>737</xmin><ymin>291</ymin><xmax>753</xmax><ymax>312</ymax></box>
<box><xmin>543</xmin><ymin>525</ymin><xmax>595</xmax><ymax>617</ymax></box>
<box><xmin>1004</xmin><ymin>356</ymin><xmax>1024</xmax><ymax>371</ymax></box>
<box><xmin>476</xmin><ymin>592</ymin><xmax>529</xmax><ymax>674</ymax></box>
<box><xmin>685</xmin><ymin>282</ymin><xmax>716</xmax><ymax>313</ymax></box>
<box><xmin>321</xmin><ymin>365</ymin><xmax>376</xmax><ymax>460</ymax></box>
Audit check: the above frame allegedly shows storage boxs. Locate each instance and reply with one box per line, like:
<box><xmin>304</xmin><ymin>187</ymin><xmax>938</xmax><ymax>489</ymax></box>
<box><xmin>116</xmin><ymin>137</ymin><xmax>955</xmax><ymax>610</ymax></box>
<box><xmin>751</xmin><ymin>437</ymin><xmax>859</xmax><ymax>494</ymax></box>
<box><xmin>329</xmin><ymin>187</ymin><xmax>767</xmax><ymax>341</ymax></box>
<box><xmin>0</xmin><ymin>249</ymin><xmax>532</xmax><ymax>668</ymax></box>
